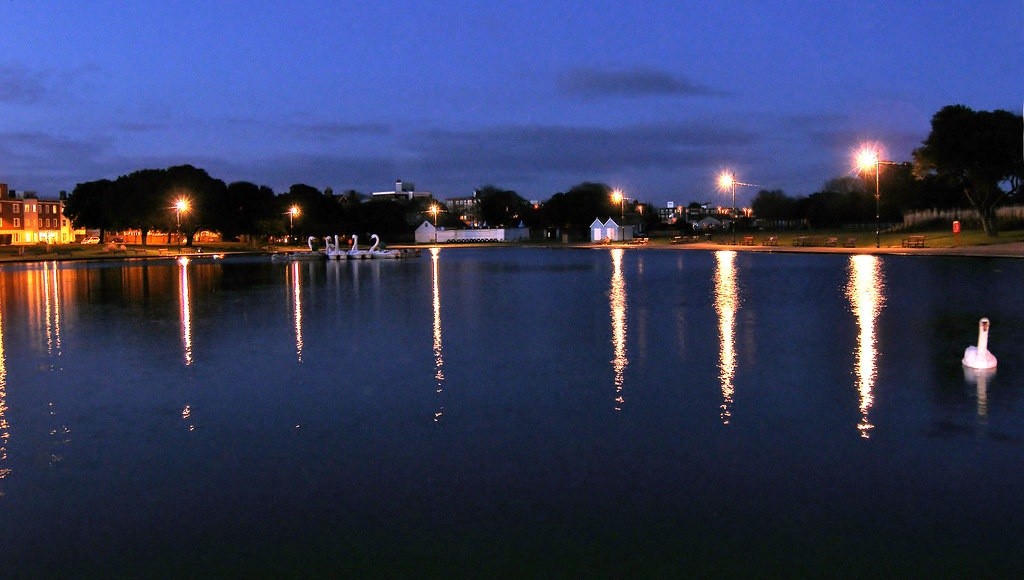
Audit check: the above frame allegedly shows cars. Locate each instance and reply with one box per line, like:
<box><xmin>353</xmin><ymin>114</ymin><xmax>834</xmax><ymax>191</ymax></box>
<box><xmin>109</xmin><ymin>238</ymin><xmax>124</xmax><ymax>243</ymax></box>
<box><xmin>81</xmin><ymin>237</ymin><xmax>100</xmax><ymax>244</ymax></box>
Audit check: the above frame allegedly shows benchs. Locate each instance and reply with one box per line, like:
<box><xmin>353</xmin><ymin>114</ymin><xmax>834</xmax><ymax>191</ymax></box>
<box><xmin>600</xmin><ymin>239</ymin><xmax>612</xmax><ymax>245</ymax></box>
<box><xmin>762</xmin><ymin>236</ymin><xmax>778</xmax><ymax>246</ymax></box>
<box><xmin>158</xmin><ymin>249</ymin><xmax>170</xmax><ymax>256</ymax></box>
<box><xmin>622</xmin><ymin>237</ymin><xmax>650</xmax><ymax>246</ymax></box>
<box><xmin>669</xmin><ymin>236</ymin><xmax>681</xmax><ymax>244</ymax></box>
<box><xmin>792</xmin><ymin>236</ymin><xmax>857</xmax><ymax>247</ymax></box>
<box><xmin>901</xmin><ymin>235</ymin><xmax>926</xmax><ymax>248</ymax></box>
<box><xmin>135</xmin><ymin>249</ymin><xmax>148</xmax><ymax>255</ymax></box>
<box><xmin>180</xmin><ymin>248</ymin><xmax>192</xmax><ymax>254</ymax></box>
<box><xmin>738</xmin><ymin>236</ymin><xmax>753</xmax><ymax>245</ymax></box>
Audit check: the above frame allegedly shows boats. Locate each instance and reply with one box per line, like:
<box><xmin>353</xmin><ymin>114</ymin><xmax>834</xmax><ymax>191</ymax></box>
<box><xmin>369</xmin><ymin>234</ymin><xmax>422</xmax><ymax>258</ymax></box>
<box><xmin>347</xmin><ymin>235</ymin><xmax>371</xmax><ymax>259</ymax></box>
<box><xmin>326</xmin><ymin>235</ymin><xmax>348</xmax><ymax>260</ymax></box>
<box><xmin>289</xmin><ymin>236</ymin><xmax>332</xmax><ymax>261</ymax></box>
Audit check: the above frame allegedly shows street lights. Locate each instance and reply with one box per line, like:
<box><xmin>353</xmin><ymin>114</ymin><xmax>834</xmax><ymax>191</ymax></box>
<box><xmin>177</xmin><ymin>202</ymin><xmax>184</xmax><ymax>254</ymax></box>
<box><xmin>617</xmin><ymin>196</ymin><xmax>625</xmax><ymax>245</ymax></box>
<box><xmin>725</xmin><ymin>174</ymin><xmax>735</xmax><ymax>246</ymax></box>
<box><xmin>866</xmin><ymin>151</ymin><xmax>880</xmax><ymax>248</ymax></box>
<box><xmin>291</xmin><ymin>208</ymin><xmax>297</xmax><ymax>246</ymax></box>
<box><xmin>433</xmin><ymin>207</ymin><xmax>437</xmax><ymax>245</ymax></box>
